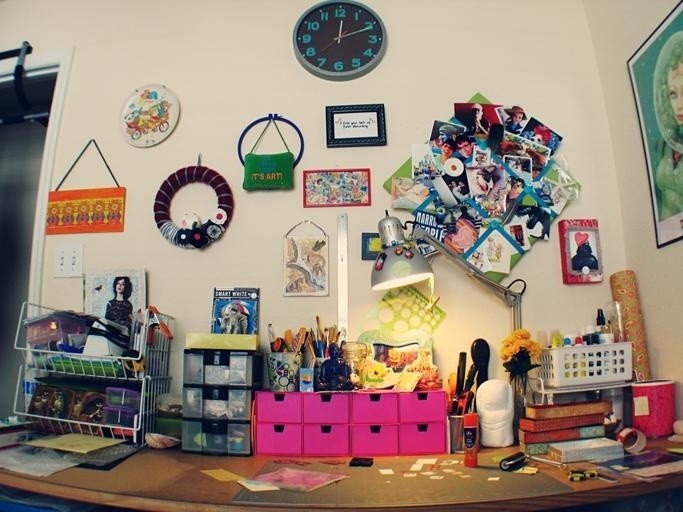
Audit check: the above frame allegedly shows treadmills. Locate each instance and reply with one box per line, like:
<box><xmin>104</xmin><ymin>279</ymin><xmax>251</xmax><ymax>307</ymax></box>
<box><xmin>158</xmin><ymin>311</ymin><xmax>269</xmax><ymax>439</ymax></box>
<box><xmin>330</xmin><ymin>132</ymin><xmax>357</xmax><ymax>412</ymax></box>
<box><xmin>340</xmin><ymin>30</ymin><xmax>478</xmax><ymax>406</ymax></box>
<box><xmin>337</xmin><ymin>213</ymin><xmax>349</xmax><ymax>349</ymax></box>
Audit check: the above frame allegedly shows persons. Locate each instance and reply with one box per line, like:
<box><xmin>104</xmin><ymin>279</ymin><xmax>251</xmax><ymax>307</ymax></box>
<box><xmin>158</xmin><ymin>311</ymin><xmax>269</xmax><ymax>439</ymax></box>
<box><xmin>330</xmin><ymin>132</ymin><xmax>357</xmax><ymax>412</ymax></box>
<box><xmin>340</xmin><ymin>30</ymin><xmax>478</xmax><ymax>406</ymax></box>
<box><xmin>455</xmin><ymin>135</ymin><xmax>477</xmax><ymax>163</ymax></box>
<box><xmin>653</xmin><ymin>30</ymin><xmax>682</xmax><ymax>219</ymax></box>
<box><xmin>104</xmin><ymin>276</ymin><xmax>132</xmax><ymax>331</ymax></box>
<box><xmin>465</xmin><ymin>103</ymin><xmax>490</xmax><ymax>135</ymax></box>
<box><xmin>503</xmin><ymin>105</ymin><xmax>528</xmax><ymax>132</ymax></box>
<box><xmin>434</xmin><ymin>138</ymin><xmax>455</xmax><ymax>174</ymax></box>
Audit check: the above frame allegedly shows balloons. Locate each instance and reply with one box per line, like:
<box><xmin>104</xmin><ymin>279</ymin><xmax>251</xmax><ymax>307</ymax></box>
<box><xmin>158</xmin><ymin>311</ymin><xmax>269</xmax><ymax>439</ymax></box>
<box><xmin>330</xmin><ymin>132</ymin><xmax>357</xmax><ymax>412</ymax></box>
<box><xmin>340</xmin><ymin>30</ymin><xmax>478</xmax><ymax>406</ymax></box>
<box><xmin>293</xmin><ymin>0</ymin><xmax>389</xmax><ymax>80</ymax></box>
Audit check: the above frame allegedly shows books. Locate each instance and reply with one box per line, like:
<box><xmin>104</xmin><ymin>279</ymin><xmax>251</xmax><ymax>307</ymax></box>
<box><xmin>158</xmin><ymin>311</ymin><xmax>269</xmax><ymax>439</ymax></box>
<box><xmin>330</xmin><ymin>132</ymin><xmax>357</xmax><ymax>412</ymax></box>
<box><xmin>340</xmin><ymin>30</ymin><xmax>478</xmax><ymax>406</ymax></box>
<box><xmin>181</xmin><ymin>348</ymin><xmax>448</xmax><ymax>457</ymax></box>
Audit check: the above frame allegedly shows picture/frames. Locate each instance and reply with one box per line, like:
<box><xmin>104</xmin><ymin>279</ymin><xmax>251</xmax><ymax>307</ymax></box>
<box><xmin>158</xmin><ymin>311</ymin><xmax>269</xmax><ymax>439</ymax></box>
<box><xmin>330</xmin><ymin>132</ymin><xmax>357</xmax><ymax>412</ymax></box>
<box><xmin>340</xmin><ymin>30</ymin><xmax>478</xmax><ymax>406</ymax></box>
<box><xmin>0</xmin><ymin>420</ymin><xmax>682</xmax><ymax>512</ymax></box>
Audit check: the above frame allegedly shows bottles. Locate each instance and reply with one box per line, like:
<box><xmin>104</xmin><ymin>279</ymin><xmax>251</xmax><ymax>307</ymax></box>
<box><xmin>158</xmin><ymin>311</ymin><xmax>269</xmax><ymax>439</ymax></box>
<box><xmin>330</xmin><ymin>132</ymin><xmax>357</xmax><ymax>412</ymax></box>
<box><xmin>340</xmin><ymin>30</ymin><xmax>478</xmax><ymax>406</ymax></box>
<box><xmin>504</xmin><ymin>106</ymin><xmax>527</xmax><ymax>120</ymax></box>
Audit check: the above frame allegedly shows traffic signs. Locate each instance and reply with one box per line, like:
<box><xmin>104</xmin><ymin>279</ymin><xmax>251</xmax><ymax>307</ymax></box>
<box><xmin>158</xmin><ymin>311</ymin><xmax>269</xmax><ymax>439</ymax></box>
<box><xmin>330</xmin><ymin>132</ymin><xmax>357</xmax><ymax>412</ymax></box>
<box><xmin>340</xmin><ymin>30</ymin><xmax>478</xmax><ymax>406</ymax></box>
<box><xmin>55</xmin><ymin>246</ymin><xmax>86</xmax><ymax>278</ymax></box>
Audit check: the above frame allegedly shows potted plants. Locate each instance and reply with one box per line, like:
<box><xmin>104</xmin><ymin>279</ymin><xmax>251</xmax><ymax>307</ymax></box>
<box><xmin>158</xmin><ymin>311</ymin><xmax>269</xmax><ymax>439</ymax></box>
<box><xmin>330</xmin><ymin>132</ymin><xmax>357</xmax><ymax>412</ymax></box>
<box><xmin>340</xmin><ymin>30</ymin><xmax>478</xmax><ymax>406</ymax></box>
<box><xmin>627</xmin><ymin>0</ymin><xmax>683</xmax><ymax>249</ymax></box>
<box><xmin>303</xmin><ymin>167</ymin><xmax>373</xmax><ymax>207</ymax></box>
<box><xmin>326</xmin><ymin>104</ymin><xmax>389</xmax><ymax>147</ymax></box>
<box><xmin>561</xmin><ymin>215</ymin><xmax>605</xmax><ymax>285</ymax></box>
<box><xmin>283</xmin><ymin>219</ymin><xmax>330</xmax><ymax>297</ymax></box>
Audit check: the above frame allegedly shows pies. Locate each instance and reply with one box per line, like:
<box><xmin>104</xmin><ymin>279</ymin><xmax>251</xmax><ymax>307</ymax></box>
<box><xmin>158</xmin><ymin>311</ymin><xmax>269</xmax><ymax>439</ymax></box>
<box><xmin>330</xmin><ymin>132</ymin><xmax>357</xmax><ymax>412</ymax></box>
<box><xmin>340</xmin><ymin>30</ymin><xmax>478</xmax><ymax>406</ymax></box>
<box><xmin>306</xmin><ymin>316</ymin><xmax>346</xmax><ymax>359</ymax></box>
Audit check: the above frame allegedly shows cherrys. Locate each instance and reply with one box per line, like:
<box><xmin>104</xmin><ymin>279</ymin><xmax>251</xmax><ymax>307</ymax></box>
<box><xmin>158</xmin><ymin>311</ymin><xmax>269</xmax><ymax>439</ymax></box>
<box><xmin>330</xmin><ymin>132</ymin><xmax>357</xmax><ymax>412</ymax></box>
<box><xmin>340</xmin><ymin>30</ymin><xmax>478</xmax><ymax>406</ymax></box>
<box><xmin>500</xmin><ymin>452</ymin><xmax>568</xmax><ymax>471</ymax></box>
<box><xmin>305</xmin><ymin>316</ymin><xmax>343</xmax><ymax>357</ymax></box>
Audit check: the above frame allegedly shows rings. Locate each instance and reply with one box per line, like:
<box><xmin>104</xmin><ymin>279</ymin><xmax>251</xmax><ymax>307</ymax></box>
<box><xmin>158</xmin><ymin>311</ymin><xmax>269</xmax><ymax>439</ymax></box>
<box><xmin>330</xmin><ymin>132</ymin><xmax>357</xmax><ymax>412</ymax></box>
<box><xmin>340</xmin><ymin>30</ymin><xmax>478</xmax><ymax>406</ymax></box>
<box><xmin>268</xmin><ymin>324</ymin><xmax>306</xmax><ymax>353</ymax></box>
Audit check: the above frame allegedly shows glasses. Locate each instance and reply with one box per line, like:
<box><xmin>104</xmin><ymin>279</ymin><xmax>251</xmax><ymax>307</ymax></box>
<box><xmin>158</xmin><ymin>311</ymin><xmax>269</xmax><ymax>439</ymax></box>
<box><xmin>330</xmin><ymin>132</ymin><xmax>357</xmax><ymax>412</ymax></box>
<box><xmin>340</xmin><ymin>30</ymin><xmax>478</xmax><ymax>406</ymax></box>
<box><xmin>371</xmin><ymin>210</ymin><xmax>527</xmax><ymax>395</ymax></box>
